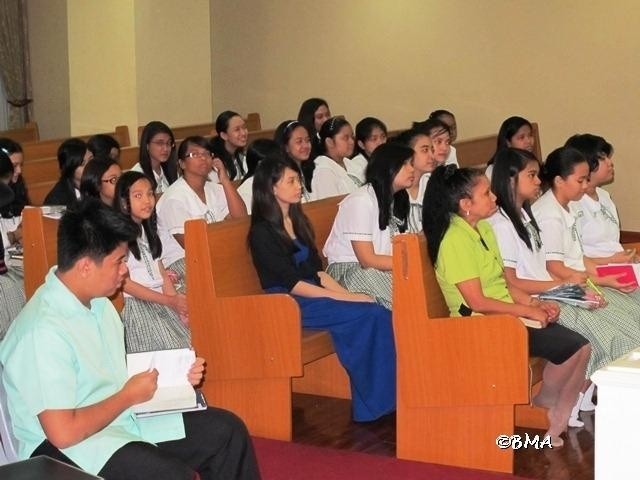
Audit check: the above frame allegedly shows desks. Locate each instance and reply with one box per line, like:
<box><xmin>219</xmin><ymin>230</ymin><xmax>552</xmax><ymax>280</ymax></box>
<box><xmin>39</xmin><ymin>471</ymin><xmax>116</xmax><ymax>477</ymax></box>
<box><xmin>589</xmin><ymin>353</ymin><xmax>640</xmax><ymax>480</ymax></box>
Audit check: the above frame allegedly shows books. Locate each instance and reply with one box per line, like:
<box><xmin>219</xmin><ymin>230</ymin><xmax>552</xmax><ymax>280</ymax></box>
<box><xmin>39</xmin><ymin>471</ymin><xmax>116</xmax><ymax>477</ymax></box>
<box><xmin>528</xmin><ymin>281</ymin><xmax>599</xmax><ymax>313</ymax></box>
<box><xmin>514</xmin><ymin>316</ymin><xmax>542</xmax><ymax>331</ymax></box>
<box><xmin>124</xmin><ymin>348</ymin><xmax>209</xmax><ymax>418</ymax></box>
<box><xmin>595</xmin><ymin>263</ymin><xmax>640</xmax><ymax>290</ymax></box>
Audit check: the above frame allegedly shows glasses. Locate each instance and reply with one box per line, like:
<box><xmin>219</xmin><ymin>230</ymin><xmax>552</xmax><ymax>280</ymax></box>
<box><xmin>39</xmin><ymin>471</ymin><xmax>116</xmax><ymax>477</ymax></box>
<box><xmin>148</xmin><ymin>139</ymin><xmax>176</xmax><ymax>147</ymax></box>
<box><xmin>182</xmin><ymin>149</ymin><xmax>214</xmax><ymax>161</ymax></box>
<box><xmin>99</xmin><ymin>175</ymin><xmax>119</xmax><ymax>186</ymax></box>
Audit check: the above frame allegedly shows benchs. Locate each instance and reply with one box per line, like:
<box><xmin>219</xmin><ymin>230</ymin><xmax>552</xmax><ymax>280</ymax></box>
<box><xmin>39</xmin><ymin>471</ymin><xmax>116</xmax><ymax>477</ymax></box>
<box><xmin>137</xmin><ymin>112</ymin><xmax>262</xmax><ymax>145</ymax></box>
<box><xmin>20</xmin><ymin>125</ymin><xmax>131</xmax><ymax>161</ymax></box>
<box><xmin>21</xmin><ymin>193</ymin><xmax>164</xmax><ymax>308</ymax></box>
<box><xmin>451</xmin><ymin>124</ymin><xmax>546</xmax><ymax>168</ymax></box>
<box><xmin>390</xmin><ymin>232</ymin><xmax>639</xmax><ymax>476</ymax></box>
<box><xmin>0</xmin><ymin>122</ymin><xmax>40</xmax><ymax>146</ymax></box>
<box><xmin>24</xmin><ymin>180</ymin><xmax>58</xmax><ymax>205</ymax></box>
<box><xmin>182</xmin><ymin>191</ymin><xmax>354</xmax><ymax>445</ymax></box>
<box><xmin>22</xmin><ymin>144</ymin><xmax>142</xmax><ymax>184</ymax></box>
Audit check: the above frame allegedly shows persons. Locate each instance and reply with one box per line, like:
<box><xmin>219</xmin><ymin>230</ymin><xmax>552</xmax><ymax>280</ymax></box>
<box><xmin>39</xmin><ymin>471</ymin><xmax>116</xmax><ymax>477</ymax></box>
<box><xmin>0</xmin><ymin>229</ymin><xmax>27</xmax><ymax>342</ymax></box>
<box><xmin>418</xmin><ymin>119</ymin><xmax>451</xmax><ymax>173</ymax></box>
<box><xmin>433</xmin><ymin>166</ymin><xmax>591</xmax><ymax>448</ymax></box>
<box><xmin>237</xmin><ymin>137</ymin><xmax>286</xmax><ymax>215</ymax></box>
<box><xmin>431</xmin><ymin>109</ymin><xmax>460</xmax><ymax>173</ymax></box>
<box><xmin>129</xmin><ymin>122</ymin><xmax>181</xmax><ymax>203</ymax></box>
<box><xmin>43</xmin><ymin>139</ymin><xmax>94</xmax><ymax>209</ymax></box>
<box><xmin>325</xmin><ymin>143</ymin><xmax>415</xmax><ymax>308</ymax></box>
<box><xmin>110</xmin><ymin>171</ymin><xmax>190</xmax><ymax>354</ymax></box>
<box><xmin>157</xmin><ymin>135</ymin><xmax>247</xmax><ymax>293</ymax></box>
<box><xmin>250</xmin><ymin>152</ymin><xmax>397</xmax><ymax>425</ymax></box>
<box><xmin>1</xmin><ymin>134</ymin><xmax>35</xmax><ymax>240</ymax></box>
<box><xmin>486</xmin><ymin>116</ymin><xmax>547</xmax><ymax>201</ymax></box>
<box><xmin>348</xmin><ymin>117</ymin><xmax>388</xmax><ymax>184</ymax></box>
<box><xmin>207</xmin><ymin>110</ymin><xmax>248</xmax><ymax>188</ymax></box>
<box><xmin>2</xmin><ymin>201</ymin><xmax>259</xmax><ymax>479</ymax></box>
<box><xmin>563</xmin><ymin>133</ymin><xmax>640</xmax><ymax>267</ymax></box>
<box><xmin>397</xmin><ymin>132</ymin><xmax>435</xmax><ymax>234</ymax></box>
<box><xmin>488</xmin><ymin>148</ymin><xmax>640</xmax><ymax>431</ymax></box>
<box><xmin>81</xmin><ymin>155</ymin><xmax>121</xmax><ymax>208</ymax></box>
<box><xmin>310</xmin><ymin>114</ymin><xmax>362</xmax><ymax>199</ymax></box>
<box><xmin>272</xmin><ymin>119</ymin><xmax>336</xmax><ymax>202</ymax></box>
<box><xmin>297</xmin><ymin>96</ymin><xmax>334</xmax><ymax>155</ymax></box>
<box><xmin>531</xmin><ymin>148</ymin><xmax>639</xmax><ymax>309</ymax></box>
<box><xmin>86</xmin><ymin>132</ymin><xmax>131</xmax><ymax>174</ymax></box>
<box><xmin>1</xmin><ymin>150</ymin><xmax>16</xmax><ymax>187</ymax></box>
